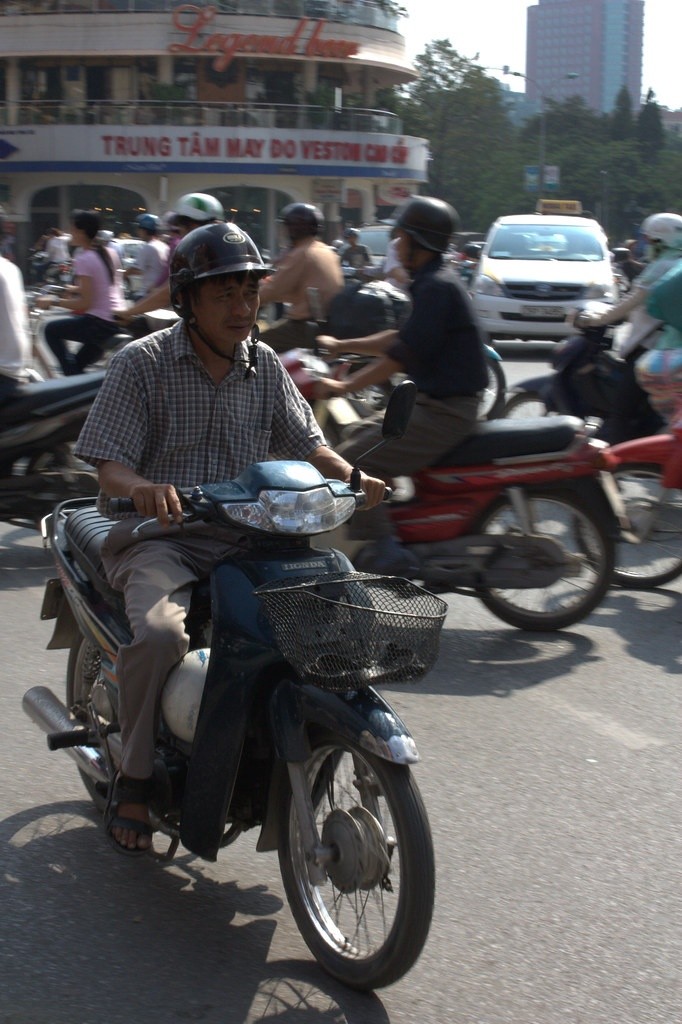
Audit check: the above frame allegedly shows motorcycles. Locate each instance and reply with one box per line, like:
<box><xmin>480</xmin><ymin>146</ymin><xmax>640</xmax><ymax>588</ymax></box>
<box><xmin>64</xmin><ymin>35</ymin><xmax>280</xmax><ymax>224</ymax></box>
<box><xmin>20</xmin><ymin>380</ymin><xmax>450</xmax><ymax>993</ymax></box>
<box><xmin>342</xmin><ymin>264</ymin><xmax>380</xmax><ymax>288</ymax></box>
<box><xmin>0</xmin><ymin>366</ymin><xmax>116</xmax><ymax>533</ymax></box>
<box><xmin>274</xmin><ymin>287</ymin><xmax>639</xmax><ymax>632</ymax></box>
<box><xmin>488</xmin><ymin>301</ymin><xmax>682</xmax><ymax>589</ymax></box>
<box><xmin>318</xmin><ymin>322</ymin><xmax>506</xmax><ymax>421</ymax></box>
<box><xmin>112</xmin><ymin>309</ymin><xmax>328</xmax><ymax>403</ymax></box>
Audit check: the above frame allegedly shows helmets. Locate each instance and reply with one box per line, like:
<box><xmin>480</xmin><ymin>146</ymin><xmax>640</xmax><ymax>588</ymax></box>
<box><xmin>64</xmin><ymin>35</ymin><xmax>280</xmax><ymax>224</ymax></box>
<box><xmin>129</xmin><ymin>214</ymin><xmax>163</xmax><ymax>232</ymax></box>
<box><xmin>273</xmin><ymin>204</ymin><xmax>326</xmax><ymax>238</ymax></box>
<box><xmin>169</xmin><ymin>222</ymin><xmax>278</xmax><ymax>299</ymax></box>
<box><xmin>462</xmin><ymin>243</ymin><xmax>482</xmax><ymax>259</ymax></box>
<box><xmin>344</xmin><ymin>227</ymin><xmax>360</xmax><ymax>238</ymax></box>
<box><xmin>379</xmin><ymin>193</ymin><xmax>460</xmax><ymax>254</ymax></box>
<box><xmin>167</xmin><ymin>193</ymin><xmax>224</xmax><ymax>229</ymax></box>
<box><xmin>160</xmin><ymin>647</ymin><xmax>211</xmax><ymax>743</ymax></box>
<box><xmin>639</xmin><ymin>212</ymin><xmax>682</xmax><ymax>251</ymax></box>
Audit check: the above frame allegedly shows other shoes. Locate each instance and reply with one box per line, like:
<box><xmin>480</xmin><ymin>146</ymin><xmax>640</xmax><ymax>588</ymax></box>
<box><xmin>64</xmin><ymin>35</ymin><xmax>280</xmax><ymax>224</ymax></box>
<box><xmin>352</xmin><ymin>546</ymin><xmax>406</xmax><ymax>576</ymax></box>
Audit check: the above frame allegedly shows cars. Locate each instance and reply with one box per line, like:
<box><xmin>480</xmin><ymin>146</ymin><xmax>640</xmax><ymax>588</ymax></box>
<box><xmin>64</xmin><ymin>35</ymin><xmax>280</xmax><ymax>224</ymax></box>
<box><xmin>351</xmin><ymin>225</ymin><xmax>397</xmax><ymax>265</ymax></box>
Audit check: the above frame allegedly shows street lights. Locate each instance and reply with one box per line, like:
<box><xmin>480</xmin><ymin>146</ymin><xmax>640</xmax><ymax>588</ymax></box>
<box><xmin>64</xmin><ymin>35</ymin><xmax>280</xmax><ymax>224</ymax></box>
<box><xmin>511</xmin><ymin>72</ymin><xmax>580</xmax><ymax>199</ymax></box>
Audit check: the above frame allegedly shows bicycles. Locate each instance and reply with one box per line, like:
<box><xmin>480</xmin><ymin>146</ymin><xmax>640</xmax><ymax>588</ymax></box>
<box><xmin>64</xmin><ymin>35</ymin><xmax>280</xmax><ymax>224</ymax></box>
<box><xmin>24</xmin><ymin>278</ymin><xmax>134</xmax><ymax>383</ymax></box>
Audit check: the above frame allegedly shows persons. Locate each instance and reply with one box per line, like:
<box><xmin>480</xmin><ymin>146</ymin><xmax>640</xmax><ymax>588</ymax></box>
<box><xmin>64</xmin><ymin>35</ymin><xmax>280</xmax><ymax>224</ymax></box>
<box><xmin>70</xmin><ymin>220</ymin><xmax>385</xmax><ymax>857</ymax></box>
<box><xmin>299</xmin><ymin>197</ymin><xmax>484</xmax><ymax>587</ymax></box>
<box><xmin>0</xmin><ymin>194</ymin><xmax>682</xmax><ymax>447</ymax></box>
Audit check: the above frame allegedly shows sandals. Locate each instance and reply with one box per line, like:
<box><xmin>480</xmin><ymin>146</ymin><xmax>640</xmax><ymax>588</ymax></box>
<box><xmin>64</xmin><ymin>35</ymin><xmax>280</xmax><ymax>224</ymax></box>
<box><xmin>103</xmin><ymin>769</ymin><xmax>154</xmax><ymax>857</ymax></box>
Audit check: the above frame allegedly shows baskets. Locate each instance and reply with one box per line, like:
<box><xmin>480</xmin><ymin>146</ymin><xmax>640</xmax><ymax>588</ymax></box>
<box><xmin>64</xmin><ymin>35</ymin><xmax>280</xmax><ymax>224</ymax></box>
<box><xmin>252</xmin><ymin>574</ymin><xmax>448</xmax><ymax>693</ymax></box>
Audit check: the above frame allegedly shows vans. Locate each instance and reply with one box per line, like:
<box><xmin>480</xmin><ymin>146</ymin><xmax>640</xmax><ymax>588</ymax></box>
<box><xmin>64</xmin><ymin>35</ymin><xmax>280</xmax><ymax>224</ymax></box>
<box><xmin>464</xmin><ymin>213</ymin><xmax>632</xmax><ymax>350</ymax></box>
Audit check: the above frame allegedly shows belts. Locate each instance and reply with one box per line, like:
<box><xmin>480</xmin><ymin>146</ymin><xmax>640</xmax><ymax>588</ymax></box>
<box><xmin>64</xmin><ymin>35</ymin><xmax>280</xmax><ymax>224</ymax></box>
<box><xmin>307</xmin><ymin>641</ymin><xmax>425</xmax><ymax>687</ymax></box>
